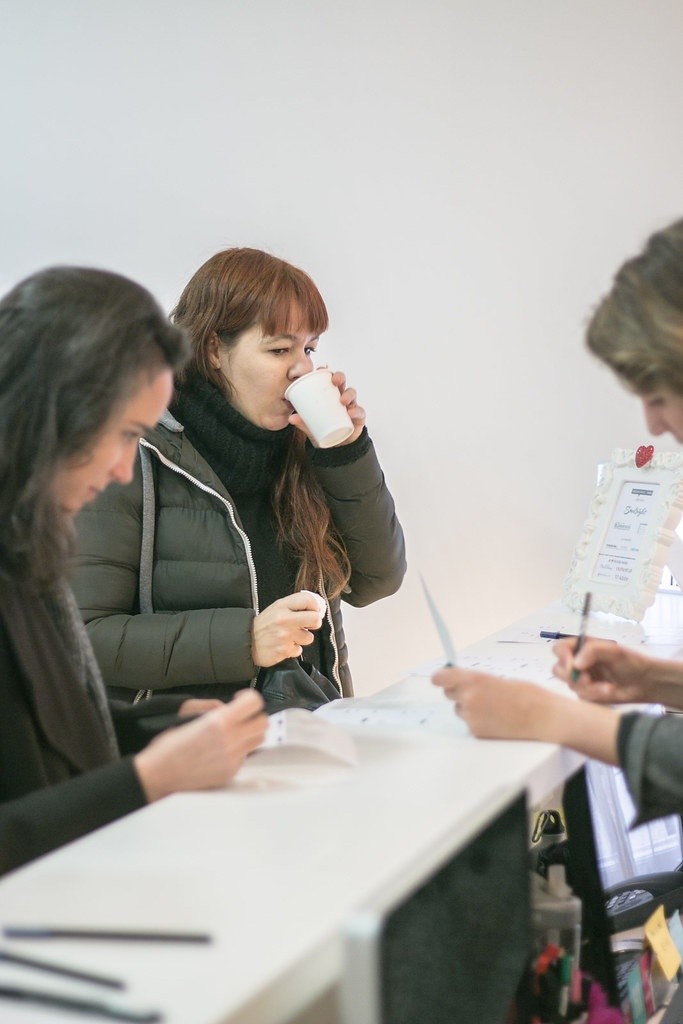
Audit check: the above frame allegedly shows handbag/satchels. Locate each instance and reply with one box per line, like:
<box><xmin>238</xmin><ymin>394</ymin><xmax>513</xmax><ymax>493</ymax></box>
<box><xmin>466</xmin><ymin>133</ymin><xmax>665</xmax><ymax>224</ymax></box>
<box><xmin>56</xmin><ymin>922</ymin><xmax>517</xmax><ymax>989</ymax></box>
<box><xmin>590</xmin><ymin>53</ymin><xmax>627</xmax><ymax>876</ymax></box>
<box><xmin>259</xmin><ymin>655</ymin><xmax>344</xmax><ymax>716</ymax></box>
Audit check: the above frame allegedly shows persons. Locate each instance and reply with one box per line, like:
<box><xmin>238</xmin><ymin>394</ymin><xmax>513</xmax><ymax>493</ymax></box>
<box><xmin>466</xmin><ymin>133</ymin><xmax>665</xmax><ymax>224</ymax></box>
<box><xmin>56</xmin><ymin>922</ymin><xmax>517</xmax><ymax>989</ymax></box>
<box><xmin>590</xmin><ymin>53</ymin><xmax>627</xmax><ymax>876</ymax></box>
<box><xmin>0</xmin><ymin>262</ymin><xmax>270</xmax><ymax>884</ymax></box>
<box><xmin>427</xmin><ymin>217</ymin><xmax>683</xmax><ymax>834</ymax></box>
<box><xmin>54</xmin><ymin>244</ymin><xmax>409</xmax><ymax>758</ymax></box>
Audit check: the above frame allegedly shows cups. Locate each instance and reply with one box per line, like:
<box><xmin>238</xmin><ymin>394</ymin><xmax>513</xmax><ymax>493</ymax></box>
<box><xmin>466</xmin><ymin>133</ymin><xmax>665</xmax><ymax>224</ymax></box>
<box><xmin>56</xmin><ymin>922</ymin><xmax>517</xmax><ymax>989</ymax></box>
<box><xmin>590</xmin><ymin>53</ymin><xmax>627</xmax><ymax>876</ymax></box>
<box><xmin>284</xmin><ymin>369</ymin><xmax>354</xmax><ymax>448</ymax></box>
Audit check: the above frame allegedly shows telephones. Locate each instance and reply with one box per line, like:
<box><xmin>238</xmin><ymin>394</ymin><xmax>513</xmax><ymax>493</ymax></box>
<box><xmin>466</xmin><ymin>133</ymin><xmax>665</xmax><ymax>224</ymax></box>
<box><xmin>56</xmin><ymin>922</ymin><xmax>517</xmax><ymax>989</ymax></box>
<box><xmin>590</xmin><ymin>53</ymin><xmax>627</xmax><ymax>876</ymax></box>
<box><xmin>601</xmin><ymin>868</ymin><xmax>683</xmax><ymax>936</ymax></box>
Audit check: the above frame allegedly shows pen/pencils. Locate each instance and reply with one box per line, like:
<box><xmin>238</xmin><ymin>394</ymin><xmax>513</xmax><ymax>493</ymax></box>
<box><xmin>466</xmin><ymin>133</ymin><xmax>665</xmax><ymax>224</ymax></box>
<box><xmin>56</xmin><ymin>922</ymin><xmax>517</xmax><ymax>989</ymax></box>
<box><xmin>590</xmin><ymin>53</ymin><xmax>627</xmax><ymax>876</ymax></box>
<box><xmin>534</xmin><ymin>942</ymin><xmax>595</xmax><ymax>1023</ymax></box>
<box><xmin>539</xmin><ymin>629</ymin><xmax>617</xmax><ymax>645</ymax></box>
<box><xmin>136</xmin><ymin>711</ymin><xmax>203</xmax><ymax>731</ymax></box>
<box><xmin>570</xmin><ymin>590</ymin><xmax>593</xmax><ymax>686</ymax></box>
